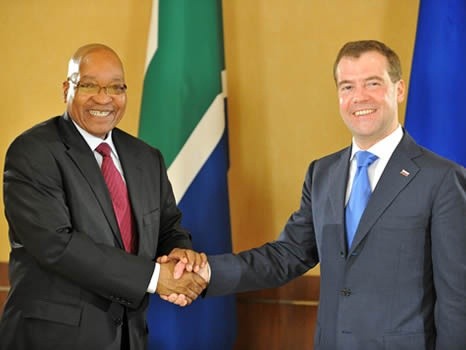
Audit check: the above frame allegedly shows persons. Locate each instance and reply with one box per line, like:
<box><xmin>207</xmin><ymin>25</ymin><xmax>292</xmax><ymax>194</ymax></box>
<box><xmin>137</xmin><ymin>0</ymin><xmax>242</xmax><ymax>349</ymax></box>
<box><xmin>155</xmin><ymin>40</ymin><xmax>466</xmax><ymax>350</ymax></box>
<box><xmin>403</xmin><ymin>0</ymin><xmax>466</xmax><ymax>169</ymax></box>
<box><xmin>0</xmin><ymin>43</ymin><xmax>206</xmax><ymax>350</ymax></box>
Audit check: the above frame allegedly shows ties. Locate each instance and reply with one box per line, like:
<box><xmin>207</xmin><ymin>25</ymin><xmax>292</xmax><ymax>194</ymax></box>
<box><xmin>95</xmin><ymin>142</ymin><xmax>138</xmax><ymax>257</ymax></box>
<box><xmin>344</xmin><ymin>151</ymin><xmax>379</xmax><ymax>256</ymax></box>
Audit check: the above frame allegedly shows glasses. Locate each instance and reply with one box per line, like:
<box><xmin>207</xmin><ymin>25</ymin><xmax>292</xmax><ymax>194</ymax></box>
<box><xmin>67</xmin><ymin>78</ymin><xmax>127</xmax><ymax>95</ymax></box>
